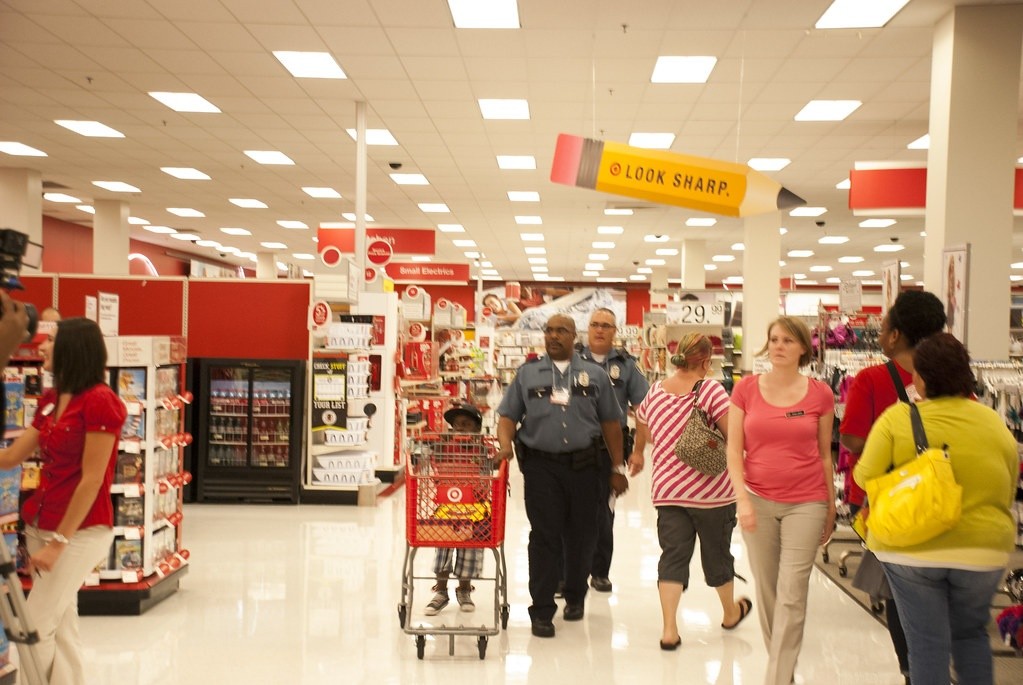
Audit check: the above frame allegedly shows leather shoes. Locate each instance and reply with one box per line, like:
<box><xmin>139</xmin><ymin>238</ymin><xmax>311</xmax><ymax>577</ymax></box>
<box><xmin>590</xmin><ymin>576</ymin><xmax>612</xmax><ymax>592</ymax></box>
<box><xmin>554</xmin><ymin>579</ymin><xmax>565</xmax><ymax>598</ymax></box>
<box><xmin>531</xmin><ymin>615</ymin><xmax>555</xmax><ymax>637</ymax></box>
<box><xmin>563</xmin><ymin>602</ymin><xmax>583</xmax><ymax>620</ymax></box>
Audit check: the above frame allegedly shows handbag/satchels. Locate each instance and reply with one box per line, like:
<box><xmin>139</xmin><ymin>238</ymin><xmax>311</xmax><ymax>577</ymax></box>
<box><xmin>865</xmin><ymin>402</ymin><xmax>961</xmax><ymax>547</ymax></box>
<box><xmin>674</xmin><ymin>379</ymin><xmax>728</xmax><ymax>476</ymax></box>
<box><xmin>485</xmin><ymin>378</ymin><xmax>505</xmax><ymax>412</ymax></box>
<box><xmin>825</xmin><ymin>321</ymin><xmax>885</xmax><ymax>350</ymax></box>
<box><xmin>621</xmin><ymin>425</ymin><xmax>635</xmax><ymax>459</ymax></box>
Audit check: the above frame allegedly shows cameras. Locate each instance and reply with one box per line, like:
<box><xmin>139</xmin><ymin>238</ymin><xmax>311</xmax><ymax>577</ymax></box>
<box><xmin>0</xmin><ymin>228</ymin><xmax>38</xmax><ymax>344</ymax></box>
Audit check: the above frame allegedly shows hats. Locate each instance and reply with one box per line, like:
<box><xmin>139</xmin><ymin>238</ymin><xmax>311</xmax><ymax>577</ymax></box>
<box><xmin>444</xmin><ymin>404</ymin><xmax>483</xmax><ymax>425</ymax></box>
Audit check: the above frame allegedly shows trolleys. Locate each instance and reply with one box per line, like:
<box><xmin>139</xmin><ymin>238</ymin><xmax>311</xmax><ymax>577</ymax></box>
<box><xmin>400</xmin><ymin>430</ymin><xmax>514</xmax><ymax>658</ymax></box>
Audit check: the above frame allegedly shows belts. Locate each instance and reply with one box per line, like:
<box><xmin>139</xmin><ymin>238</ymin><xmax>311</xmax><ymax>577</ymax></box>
<box><xmin>523</xmin><ymin>446</ymin><xmax>597</xmax><ymax>461</ymax></box>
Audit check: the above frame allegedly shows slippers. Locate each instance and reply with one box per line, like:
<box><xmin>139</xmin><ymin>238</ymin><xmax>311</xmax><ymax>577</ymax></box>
<box><xmin>721</xmin><ymin>599</ymin><xmax>754</xmax><ymax>629</ymax></box>
<box><xmin>660</xmin><ymin>634</ymin><xmax>682</xmax><ymax>650</ymax></box>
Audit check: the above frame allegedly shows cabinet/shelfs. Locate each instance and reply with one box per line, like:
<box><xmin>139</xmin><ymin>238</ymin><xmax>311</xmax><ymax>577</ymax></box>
<box><xmin>311</xmin><ymin>278</ymin><xmax>1023</xmax><ymax>685</ymax></box>
<box><xmin>8</xmin><ymin>318</ymin><xmax>193</xmax><ymax>617</ymax></box>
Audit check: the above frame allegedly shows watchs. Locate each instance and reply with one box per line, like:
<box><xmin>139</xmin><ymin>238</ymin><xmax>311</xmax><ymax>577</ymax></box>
<box><xmin>612</xmin><ymin>464</ymin><xmax>627</xmax><ymax>475</ymax></box>
<box><xmin>50</xmin><ymin>531</ymin><xmax>70</xmax><ymax>545</ymax></box>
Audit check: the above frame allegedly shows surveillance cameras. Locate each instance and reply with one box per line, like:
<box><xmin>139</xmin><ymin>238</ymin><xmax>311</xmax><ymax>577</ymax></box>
<box><xmin>220</xmin><ymin>254</ymin><xmax>225</xmax><ymax>257</ymax></box>
<box><xmin>891</xmin><ymin>237</ymin><xmax>898</xmax><ymax>242</ymax></box>
<box><xmin>816</xmin><ymin>221</ymin><xmax>825</xmax><ymax>227</ymax></box>
<box><xmin>656</xmin><ymin>235</ymin><xmax>661</xmax><ymax>238</ymax></box>
<box><xmin>191</xmin><ymin>240</ymin><xmax>196</xmax><ymax>243</ymax></box>
<box><xmin>390</xmin><ymin>164</ymin><xmax>401</xmax><ymax>170</ymax></box>
<box><xmin>634</xmin><ymin>261</ymin><xmax>639</xmax><ymax>264</ymax></box>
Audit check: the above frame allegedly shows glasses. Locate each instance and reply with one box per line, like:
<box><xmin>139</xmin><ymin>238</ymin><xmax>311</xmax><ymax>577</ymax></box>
<box><xmin>708</xmin><ymin>360</ymin><xmax>712</xmax><ymax>367</ymax></box>
<box><xmin>589</xmin><ymin>322</ymin><xmax>615</xmax><ymax>330</ymax></box>
<box><xmin>543</xmin><ymin>326</ymin><xmax>574</xmax><ymax>336</ymax></box>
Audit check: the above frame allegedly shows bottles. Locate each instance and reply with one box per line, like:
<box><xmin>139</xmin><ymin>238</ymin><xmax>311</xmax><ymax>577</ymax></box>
<box><xmin>210</xmin><ymin>445</ymin><xmax>289</xmax><ymax>465</ymax></box>
<box><xmin>210</xmin><ymin>417</ymin><xmax>290</xmax><ymax>442</ymax></box>
<box><xmin>211</xmin><ymin>389</ymin><xmax>290</xmax><ymax>414</ymax></box>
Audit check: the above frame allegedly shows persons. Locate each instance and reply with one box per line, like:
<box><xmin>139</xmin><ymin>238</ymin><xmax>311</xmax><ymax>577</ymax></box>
<box><xmin>484</xmin><ymin>295</ymin><xmax>521</xmax><ymax>325</ymax></box>
<box><xmin>837</xmin><ymin>289</ymin><xmax>1020</xmax><ymax>685</ymax></box>
<box><xmin>727</xmin><ymin>316</ymin><xmax>836</xmax><ymax>685</ymax></box>
<box><xmin>1</xmin><ymin>291</ymin><xmax>127</xmax><ymax>685</ymax></box>
<box><xmin>493</xmin><ymin>307</ymin><xmax>651</xmax><ymax>638</ymax></box>
<box><xmin>635</xmin><ymin>332</ymin><xmax>752</xmax><ymax>650</ymax></box>
<box><xmin>423</xmin><ymin>406</ymin><xmax>499</xmax><ymax>617</ymax></box>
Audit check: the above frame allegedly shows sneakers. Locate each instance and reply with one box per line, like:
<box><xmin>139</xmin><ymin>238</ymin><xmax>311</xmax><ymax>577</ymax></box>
<box><xmin>455</xmin><ymin>585</ymin><xmax>476</xmax><ymax>612</ymax></box>
<box><xmin>424</xmin><ymin>589</ymin><xmax>449</xmax><ymax>615</ymax></box>
